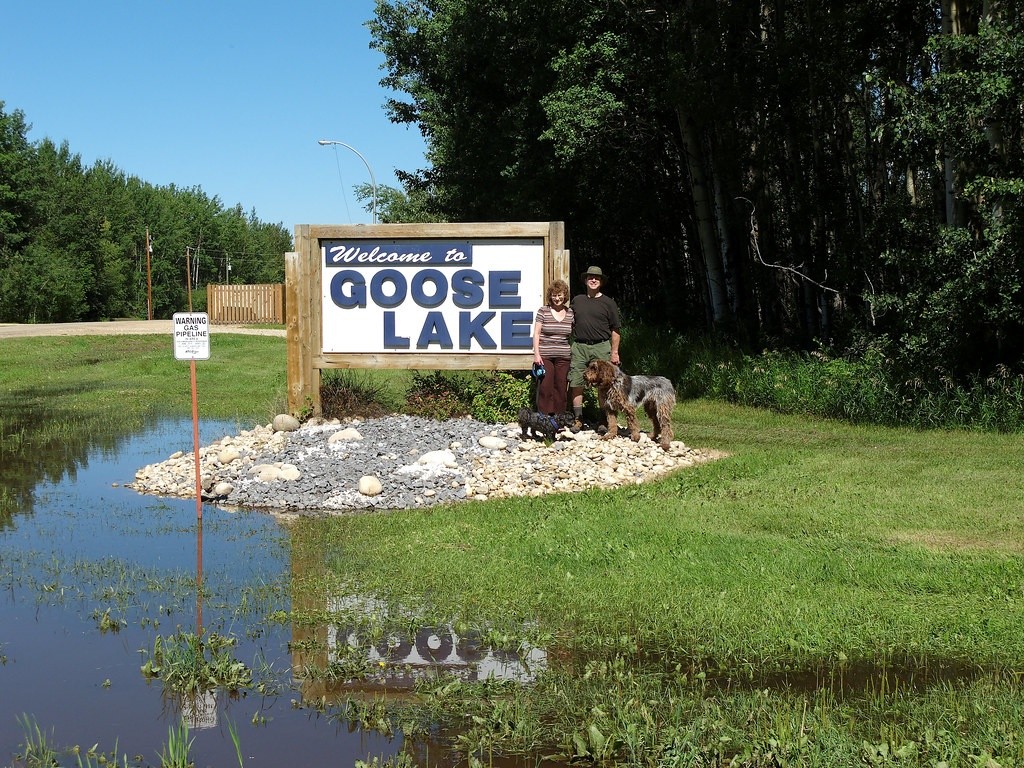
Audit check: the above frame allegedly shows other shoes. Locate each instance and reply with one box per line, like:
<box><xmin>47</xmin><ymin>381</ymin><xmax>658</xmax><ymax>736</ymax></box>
<box><xmin>569</xmin><ymin>419</ymin><xmax>583</xmax><ymax>433</ymax></box>
<box><xmin>598</xmin><ymin>420</ymin><xmax>608</xmax><ymax>435</ymax></box>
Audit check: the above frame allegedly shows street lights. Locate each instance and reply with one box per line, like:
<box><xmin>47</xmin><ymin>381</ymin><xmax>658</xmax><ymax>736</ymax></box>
<box><xmin>317</xmin><ymin>139</ymin><xmax>377</xmax><ymax>224</ymax></box>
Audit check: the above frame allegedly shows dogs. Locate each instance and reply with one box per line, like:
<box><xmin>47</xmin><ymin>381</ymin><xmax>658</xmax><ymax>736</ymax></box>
<box><xmin>583</xmin><ymin>357</ymin><xmax>676</xmax><ymax>451</ymax></box>
<box><xmin>518</xmin><ymin>410</ymin><xmax>581</xmax><ymax>443</ymax></box>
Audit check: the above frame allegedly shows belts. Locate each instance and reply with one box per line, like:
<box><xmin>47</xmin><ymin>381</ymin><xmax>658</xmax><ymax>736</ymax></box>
<box><xmin>575</xmin><ymin>339</ymin><xmax>608</xmax><ymax>345</ymax></box>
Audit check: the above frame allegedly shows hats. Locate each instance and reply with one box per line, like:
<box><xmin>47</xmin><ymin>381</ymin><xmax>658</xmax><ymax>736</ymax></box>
<box><xmin>581</xmin><ymin>266</ymin><xmax>607</xmax><ymax>279</ymax></box>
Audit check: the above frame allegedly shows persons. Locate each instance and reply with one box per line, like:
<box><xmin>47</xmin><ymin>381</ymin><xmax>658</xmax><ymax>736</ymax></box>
<box><xmin>569</xmin><ymin>266</ymin><xmax>621</xmax><ymax>434</ymax></box>
<box><xmin>533</xmin><ymin>279</ymin><xmax>575</xmax><ymax>415</ymax></box>
